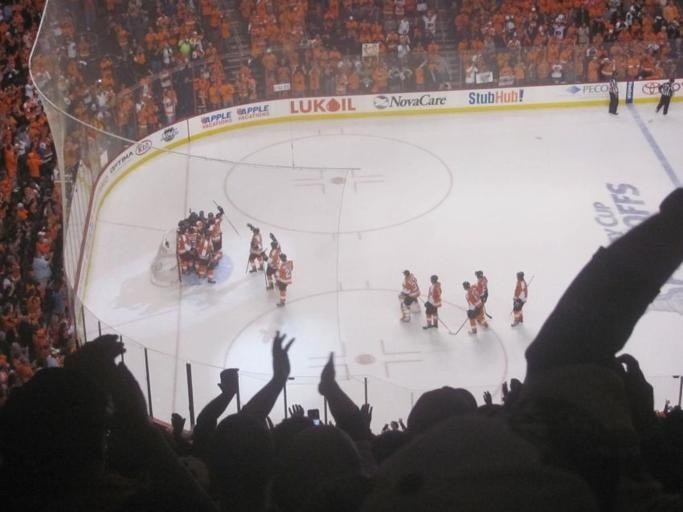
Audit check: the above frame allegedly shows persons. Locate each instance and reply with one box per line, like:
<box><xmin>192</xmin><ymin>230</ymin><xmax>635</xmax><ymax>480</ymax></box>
<box><xmin>654</xmin><ymin>76</ymin><xmax>676</xmax><ymax>116</ymax></box>
<box><xmin>0</xmin><ymin>184</ymin><xmax>682</xmax><ymax>512</ymax></box>
<box><xmin>0</xmin><ymin>0</ymin><xmax>281</xmax><ymax>183</ymax></box>
<box><xmin>282</xmin><ymin>1</ymin><xmax>682</xmax><ymax>95</ymax></box>
<box><xmin>607</xmin><ymin>70</ymin><xmax>619</xmax><ymax>116</ymax></box>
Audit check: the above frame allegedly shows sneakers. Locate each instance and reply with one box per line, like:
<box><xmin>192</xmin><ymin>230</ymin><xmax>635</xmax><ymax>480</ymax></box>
<box><xmin>483</xmin><ymin>324</ymin><xmax>488</xmax><ymax>328</ymax></box>
<box><xmin>277</xmin><ymin>303</ymin><xmax>284</xmax><ymax>306</ymax></box>
<box><xmin>182</xmin><ymin>268</ymin><xmax>216</xmax><ymax>283</ymax></box>
<box><xmin>423</xmin><ymin>324</ymin><xmax>438</xmax><ymax>329</ymax></box>
<box><xmin>511</xmin><ymin>320</ymin><xmax>522</xmax><ymax>327</ymax></box>
<box><xmin>267</xmin><ymin>286</ymin><xmax>273</xmax><ymax>290</ymax></box>
<box><xmin>249</xmin><ymin>268</ymin><xmax>263</xmax><ymax>273</ymax></box>
<box><xmin>469</xmin><ymin>330</ymin><xmax>476</xmax><ymax>335</ymax></box>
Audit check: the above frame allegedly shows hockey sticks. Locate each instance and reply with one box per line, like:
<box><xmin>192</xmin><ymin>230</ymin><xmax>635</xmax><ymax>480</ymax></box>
<box><xmin>510</xmin><ymin>272</ymin><xmax>535</xmax><ymax>315</ymax></box>
<box><xmin>283</xmin><ymin>377</ymin><xmax>295</xmax><ymax>417</ymax></box>
<box><xmin>451</xmin><ymin>319</ymin><xmax>469</xmax><ymax>335</ymax></box>
<box><xmin>189</xmin><ymin>208</ymin><xmax>192</xmax><ymax>214</ymax></box>
<box><xmin>211</xmin><ymin>199</ymin><xmax>241</xmax><ymax>237</ymax></box>
<box><xmin>429</xmin><ymin>307</ymin><xmax>455</xmax><ymax>335</ymax></box>
<box><xmin>672</xmin><ymin>375</ymin><xmax>683</xmax><ymax>407</ymax></box>
